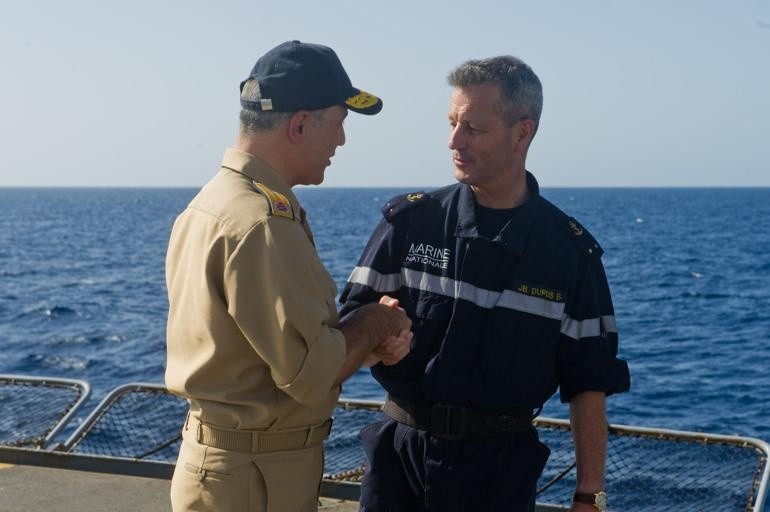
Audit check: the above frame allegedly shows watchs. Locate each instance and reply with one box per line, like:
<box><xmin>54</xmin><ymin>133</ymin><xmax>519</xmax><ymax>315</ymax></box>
<box><xmin>572</xmin><ymin>489</ymin><xmax>608</xmax><ymax>512</ymax></box>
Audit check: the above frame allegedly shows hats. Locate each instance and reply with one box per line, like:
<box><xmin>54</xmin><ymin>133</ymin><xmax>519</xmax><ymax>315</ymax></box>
<box><xmin>239</xmin><ymin>39</ymin><xmax>384</xmax><ymax>115</ymax></box>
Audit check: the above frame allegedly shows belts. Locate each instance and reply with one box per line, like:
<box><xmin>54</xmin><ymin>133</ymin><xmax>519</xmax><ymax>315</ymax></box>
<box><xmin>182</xmin><ymin>412</ymin><xmax>334</xmax><ymax>452</ymax></box>
<box><xmin>380</xmin><ymin>392</ymin><xmax>536</xmax><ymax>438</ymax></box>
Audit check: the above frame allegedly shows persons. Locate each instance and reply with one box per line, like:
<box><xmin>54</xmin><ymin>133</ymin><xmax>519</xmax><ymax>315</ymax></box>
<box><xmin>163</xmin><ymin>40</ymin><xmax>412</xmax><ymax>510</ymax></box>
<box><xmin>338</xmin><ymin>54</ymin><xmax>628</xmax><ymax>512</ymax></box>
